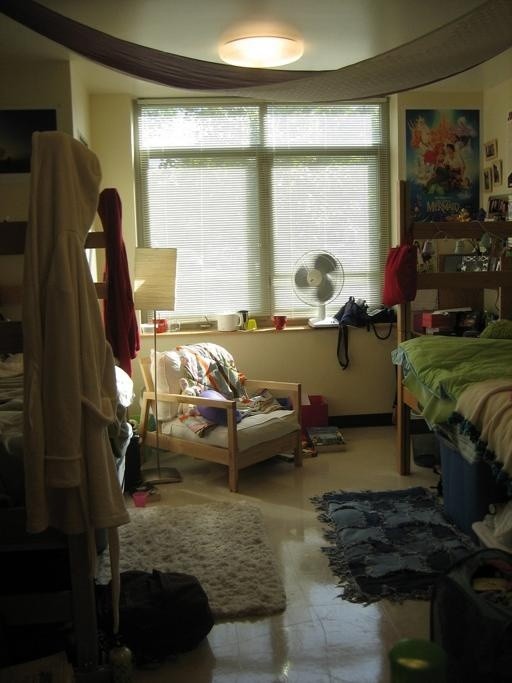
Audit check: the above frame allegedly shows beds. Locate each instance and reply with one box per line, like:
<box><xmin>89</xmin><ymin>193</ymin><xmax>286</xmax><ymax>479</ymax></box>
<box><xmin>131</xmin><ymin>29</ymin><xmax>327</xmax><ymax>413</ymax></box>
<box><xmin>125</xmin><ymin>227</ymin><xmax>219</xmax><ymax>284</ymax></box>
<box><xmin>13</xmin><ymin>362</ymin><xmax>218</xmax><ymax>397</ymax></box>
<box><xmin>398</xmin><ymin>182</ymin><xmax>511</xmax><ymax>475</ymax></box>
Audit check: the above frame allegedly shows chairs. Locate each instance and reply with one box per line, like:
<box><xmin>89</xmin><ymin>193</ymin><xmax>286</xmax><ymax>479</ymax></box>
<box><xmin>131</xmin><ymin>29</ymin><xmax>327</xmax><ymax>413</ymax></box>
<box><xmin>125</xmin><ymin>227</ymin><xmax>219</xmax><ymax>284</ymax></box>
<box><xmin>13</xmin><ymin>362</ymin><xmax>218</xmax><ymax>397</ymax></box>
<box><xmin>138</xmin><ymin>358</ymin><xmax>303</xmax><ymax>493</ymax></box>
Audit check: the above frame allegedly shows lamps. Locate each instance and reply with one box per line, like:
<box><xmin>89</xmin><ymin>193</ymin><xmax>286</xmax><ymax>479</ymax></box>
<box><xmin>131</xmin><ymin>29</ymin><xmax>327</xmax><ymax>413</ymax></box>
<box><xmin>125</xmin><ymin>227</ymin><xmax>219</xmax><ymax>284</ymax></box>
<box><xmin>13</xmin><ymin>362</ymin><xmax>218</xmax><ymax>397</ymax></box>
<box><xmin>133</xmin><ymin>247</ymin><xmax>183</xmax><ymax>484</ymax></box>
<box><xmin>219</xmin><ymin>35</ymin><xmax>304</xmax><ymax>70</ymax></box>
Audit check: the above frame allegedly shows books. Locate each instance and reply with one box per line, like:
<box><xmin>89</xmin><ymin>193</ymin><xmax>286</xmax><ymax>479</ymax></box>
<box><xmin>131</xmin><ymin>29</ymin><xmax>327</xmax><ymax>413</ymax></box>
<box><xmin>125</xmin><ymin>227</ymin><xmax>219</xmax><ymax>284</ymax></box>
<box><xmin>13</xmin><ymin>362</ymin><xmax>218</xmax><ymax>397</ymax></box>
<box><xmin>306</xmin><ymin>426</ymin><xmax>347</xmax><ymax>453</ymax></box>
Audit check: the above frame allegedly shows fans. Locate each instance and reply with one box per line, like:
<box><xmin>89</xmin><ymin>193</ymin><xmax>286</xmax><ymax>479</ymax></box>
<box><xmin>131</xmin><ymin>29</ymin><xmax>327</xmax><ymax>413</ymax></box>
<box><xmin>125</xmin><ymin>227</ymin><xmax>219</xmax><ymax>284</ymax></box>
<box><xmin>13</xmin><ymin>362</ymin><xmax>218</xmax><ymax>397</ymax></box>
<box><xmin>291</xmin><ymin>251</ymin><xmax>345</xmax><ymax>329</ymax></box>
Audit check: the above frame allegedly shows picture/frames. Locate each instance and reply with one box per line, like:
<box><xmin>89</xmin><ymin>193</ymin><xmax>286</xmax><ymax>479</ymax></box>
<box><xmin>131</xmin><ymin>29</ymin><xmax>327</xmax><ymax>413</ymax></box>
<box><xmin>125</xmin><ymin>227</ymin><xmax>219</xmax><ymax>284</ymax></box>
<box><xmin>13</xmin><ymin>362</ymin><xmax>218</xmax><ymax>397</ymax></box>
<box><xmin>481</xmin><ymin>141</ymin><xmax>511</xmax><ymax>220</ymax></box>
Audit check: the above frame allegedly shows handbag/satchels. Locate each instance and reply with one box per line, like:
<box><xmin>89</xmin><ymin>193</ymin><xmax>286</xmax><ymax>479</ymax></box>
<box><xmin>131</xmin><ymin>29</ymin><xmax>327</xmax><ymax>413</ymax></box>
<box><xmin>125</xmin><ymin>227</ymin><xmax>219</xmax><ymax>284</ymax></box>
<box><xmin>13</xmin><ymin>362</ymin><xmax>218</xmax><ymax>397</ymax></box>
<box><xmin>431</xmin><ymin>546</ymin><xmax>511</xmax><ymax>676</ymax></box>
<box><xmin>369</xmin><ymin>308</ymin><xmax>395</xmax><ymax>323</ymax></box>
<box><xmin>383</xmin><ymin>245</ymin><xmax>417</xmax><ymax>306</ymax></box>
<box><xmin>125</xmin><ymin>418</ymin><xmax>142</xmax><ymax>489</ymax></box>
<box><xmin>91</xmin><ymin>569</ymin><xmax>213</xmax><ymax>666</ymax></box>
<box><xmin>335</xmin><ymin>296</ymin><xmax>368</xmax><ymax>328</ymax></box>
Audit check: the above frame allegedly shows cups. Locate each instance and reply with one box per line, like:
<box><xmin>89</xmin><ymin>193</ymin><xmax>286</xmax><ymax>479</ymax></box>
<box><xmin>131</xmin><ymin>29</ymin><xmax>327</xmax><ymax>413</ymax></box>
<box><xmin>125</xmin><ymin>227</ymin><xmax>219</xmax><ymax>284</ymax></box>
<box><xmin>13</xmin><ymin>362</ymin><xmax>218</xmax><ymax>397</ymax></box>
<box><xmin>272</xmin><ymin>315</ymin><xmax>288</xmax><ymax>331</ymax></box>
<box><xmin>132</xmin><ymin>492</ymin><xmax>146</xmax><ymax>507</ymax></box>
<box><xmin>237</xmin><ymin>310</ymin><xmax>249</xmax><ymax>330</ymax></box>
<box><xmin>168</xmin><ymin>319</ymin><xmax>182</xmax><ymax>333</ymax></box>
<box><xmin>217</xmin><ymin>313</ymin><xmax>243</xmax><ymax>333</ymax></box>
<box><xmin>152</xmin><ymin>318</ymin><xmax>167</xmax><ymax>333</ymax></box>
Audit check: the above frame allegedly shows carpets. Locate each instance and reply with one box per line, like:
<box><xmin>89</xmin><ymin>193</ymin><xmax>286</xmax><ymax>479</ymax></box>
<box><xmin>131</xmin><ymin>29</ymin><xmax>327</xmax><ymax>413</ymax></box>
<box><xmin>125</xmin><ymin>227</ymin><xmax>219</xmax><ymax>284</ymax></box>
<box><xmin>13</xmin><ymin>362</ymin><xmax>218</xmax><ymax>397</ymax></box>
<box><xmin>310</xmin><ymin>487</ymin><xmax>487</xmax><ymax>609</ymax></box>
<box><xmin>97</xmin><ymin>502</ymin><xmax>286</xmax><ymax>623</ymax></box>
